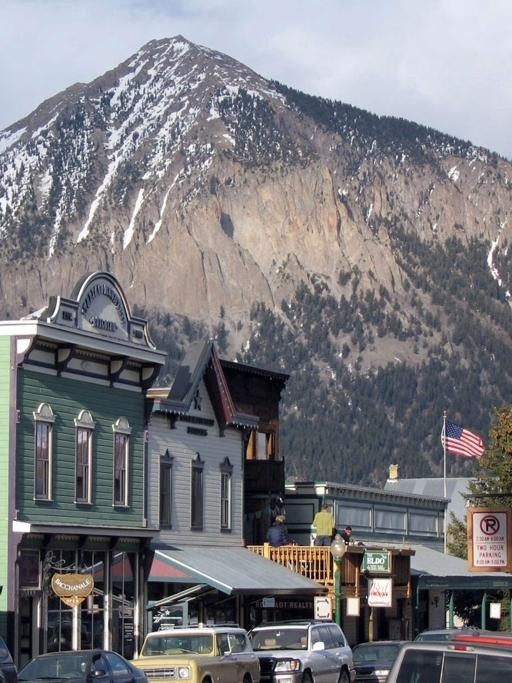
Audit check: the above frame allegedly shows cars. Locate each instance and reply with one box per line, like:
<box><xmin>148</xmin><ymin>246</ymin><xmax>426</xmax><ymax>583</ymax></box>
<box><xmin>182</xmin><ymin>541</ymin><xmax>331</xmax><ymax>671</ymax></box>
<box><xmin>352</xmin><ymin>626</ymin><xmax>512</xmax><ymax>683</ymax></box>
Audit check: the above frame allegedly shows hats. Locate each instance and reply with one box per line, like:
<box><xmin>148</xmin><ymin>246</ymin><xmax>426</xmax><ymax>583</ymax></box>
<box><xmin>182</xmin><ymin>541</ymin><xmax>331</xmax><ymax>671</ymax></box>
<box><xmin>275</xmin><ymin>515</ymin><xmax>286</xmax><ymax>523</ymax></box>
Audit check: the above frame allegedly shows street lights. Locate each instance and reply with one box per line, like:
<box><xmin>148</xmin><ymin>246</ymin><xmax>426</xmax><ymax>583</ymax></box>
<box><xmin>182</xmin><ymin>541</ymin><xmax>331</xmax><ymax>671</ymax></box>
<box><xmin>330</xmin><ymin>533</ymin><xmax>346</xmax><ymax>626</ymax></box>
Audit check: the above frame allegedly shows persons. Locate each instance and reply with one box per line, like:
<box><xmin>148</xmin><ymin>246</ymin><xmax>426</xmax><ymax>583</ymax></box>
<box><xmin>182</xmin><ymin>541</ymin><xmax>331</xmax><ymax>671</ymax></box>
<box><xmin>266</xmin><ymin>515</ymin><xmax>289</xmax><ymax>547</ymax></box>
<box><xmin>312</xmin><ymin>503</ymin><xmax>352</xmax><ymax>546</ymax></box>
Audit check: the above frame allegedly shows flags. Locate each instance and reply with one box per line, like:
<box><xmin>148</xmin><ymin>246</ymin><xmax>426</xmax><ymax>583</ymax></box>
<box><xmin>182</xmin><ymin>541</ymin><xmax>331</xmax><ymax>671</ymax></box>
<box><xmin>441</xmin><ymin>420</ymin><xmax>485</xmax><ymax>461</ymax></box>
